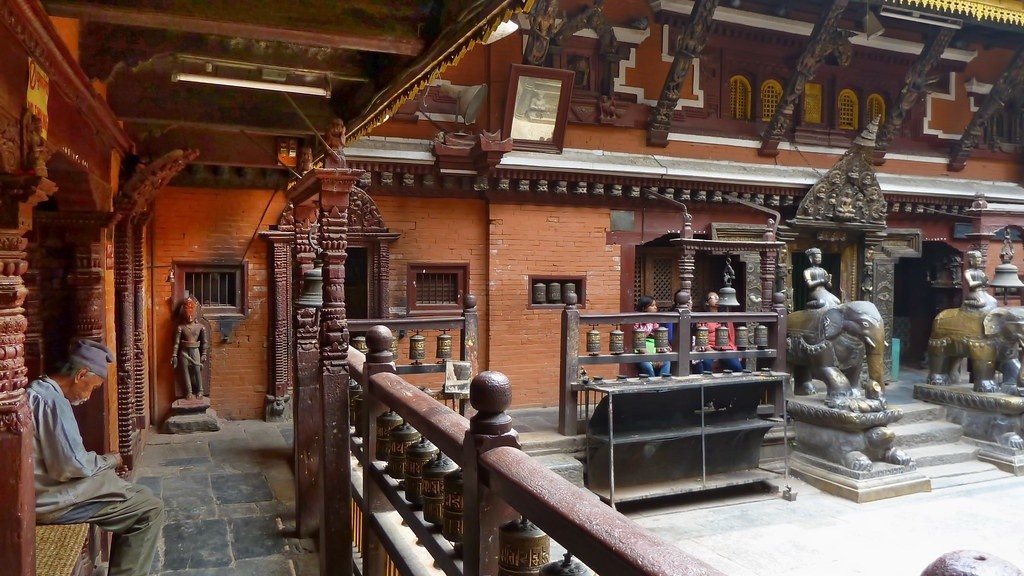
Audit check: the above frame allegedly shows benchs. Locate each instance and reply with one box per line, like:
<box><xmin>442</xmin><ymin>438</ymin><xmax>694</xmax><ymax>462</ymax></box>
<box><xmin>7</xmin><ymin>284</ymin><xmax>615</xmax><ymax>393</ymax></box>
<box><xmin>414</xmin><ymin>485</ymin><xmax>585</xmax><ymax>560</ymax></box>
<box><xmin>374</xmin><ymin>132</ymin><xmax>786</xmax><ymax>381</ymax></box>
<box><xmin>34</xmin><ymin>523</ymin><xmax>95</xmax><ymax>576</ymax></box>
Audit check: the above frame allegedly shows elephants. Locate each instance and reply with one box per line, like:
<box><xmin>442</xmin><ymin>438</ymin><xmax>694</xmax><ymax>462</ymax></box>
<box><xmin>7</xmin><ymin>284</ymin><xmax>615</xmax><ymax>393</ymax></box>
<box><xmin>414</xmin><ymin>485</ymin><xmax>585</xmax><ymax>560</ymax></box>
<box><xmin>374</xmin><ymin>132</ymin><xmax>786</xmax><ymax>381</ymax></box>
<box><xmin>784</xmin><ymin>301</ymin><xmax>884</xmax><ymax>396</ymax></box>
<box><xmin>927</xmin><ymin>307</ymin><xmax>1023</xmax><ymax>395</ymax></box>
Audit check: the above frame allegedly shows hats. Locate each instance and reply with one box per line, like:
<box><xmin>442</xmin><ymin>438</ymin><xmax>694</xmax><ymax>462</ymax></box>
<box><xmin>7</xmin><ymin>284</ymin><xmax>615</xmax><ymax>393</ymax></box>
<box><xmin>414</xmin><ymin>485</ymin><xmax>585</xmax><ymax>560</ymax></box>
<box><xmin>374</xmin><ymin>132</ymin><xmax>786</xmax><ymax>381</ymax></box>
<box><xmin>68</xmin><ymin>340</ymin><xmax>114</xmax><ymax>380</ymax></box>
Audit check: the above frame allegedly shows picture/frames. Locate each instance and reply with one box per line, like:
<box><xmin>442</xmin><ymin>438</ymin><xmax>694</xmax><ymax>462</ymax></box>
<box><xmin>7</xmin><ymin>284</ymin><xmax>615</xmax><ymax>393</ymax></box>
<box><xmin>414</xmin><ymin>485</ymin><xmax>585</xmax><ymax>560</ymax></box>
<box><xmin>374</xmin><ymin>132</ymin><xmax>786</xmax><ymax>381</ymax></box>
<box><xmin>501</xmin><ymin>64</ymin><xmax>575</xmax><ymax>156</ymax></box>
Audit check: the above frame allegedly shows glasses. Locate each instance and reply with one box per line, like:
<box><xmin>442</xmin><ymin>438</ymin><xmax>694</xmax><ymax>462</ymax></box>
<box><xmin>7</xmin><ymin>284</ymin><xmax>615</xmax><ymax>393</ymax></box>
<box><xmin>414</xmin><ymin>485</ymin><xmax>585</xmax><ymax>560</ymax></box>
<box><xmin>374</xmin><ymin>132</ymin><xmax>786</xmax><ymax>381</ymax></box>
<box><xmin>705</xmin><ymin>296</ymin><xmax>719</xmax><ymax>302</ymax></box>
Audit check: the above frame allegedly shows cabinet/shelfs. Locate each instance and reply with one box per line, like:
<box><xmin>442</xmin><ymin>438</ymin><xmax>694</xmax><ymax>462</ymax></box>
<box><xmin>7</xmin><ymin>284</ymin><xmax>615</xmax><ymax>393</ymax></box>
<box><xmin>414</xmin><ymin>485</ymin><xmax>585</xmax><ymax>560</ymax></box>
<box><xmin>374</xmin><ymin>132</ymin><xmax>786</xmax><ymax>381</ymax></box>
<box><xmin>586</xmin><ymin>372</ymin><xmax>790</xmax><ymax>510</ymax></box>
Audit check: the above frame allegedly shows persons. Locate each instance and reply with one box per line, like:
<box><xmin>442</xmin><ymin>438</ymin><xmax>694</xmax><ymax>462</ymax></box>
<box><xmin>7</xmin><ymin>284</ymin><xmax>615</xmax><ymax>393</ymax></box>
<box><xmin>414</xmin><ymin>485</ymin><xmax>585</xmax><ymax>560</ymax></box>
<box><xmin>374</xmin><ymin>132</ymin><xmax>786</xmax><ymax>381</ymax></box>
<box><xmin>26</xmin><ymin>338</ymin><xmax>163</xmax><ymax>576</ymax></box>
<box><xmin>633</xmin><ymin>289</ymin><xmax>743</xmax><ymax>378</ymax></box>
<box><xmin>964</xmin><ymin>251</ymin><xmax>998</xmax><ymax>308</ymax></box>
<box><xmin>803</xmin><ymin>247</ymin><xmax>841</xmax><ymax>309</ymax></box>
<box><xmin>170</xmin><ymin>298</ymin><xmax>208</xmax><ymax>400</ymax></box>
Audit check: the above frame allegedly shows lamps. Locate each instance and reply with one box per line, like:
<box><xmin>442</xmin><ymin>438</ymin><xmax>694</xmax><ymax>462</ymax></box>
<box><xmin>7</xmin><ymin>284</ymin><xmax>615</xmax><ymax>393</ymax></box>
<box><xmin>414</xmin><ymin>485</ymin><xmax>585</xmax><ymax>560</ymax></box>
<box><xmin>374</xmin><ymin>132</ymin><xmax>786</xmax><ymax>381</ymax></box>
<box><xmin>440</xmin><ymin>83</ymin><xmax>488</xmax><ymax>125</ymax></box>
<box><xmin>172</xmin><ymin>53</ymin><xmax>334</xmax><ymax>99</ymax></box>
<box><xmin>876</xmin><ymin>5</ymin><xmax>963</xmax><ymax>29</ymax></box>
